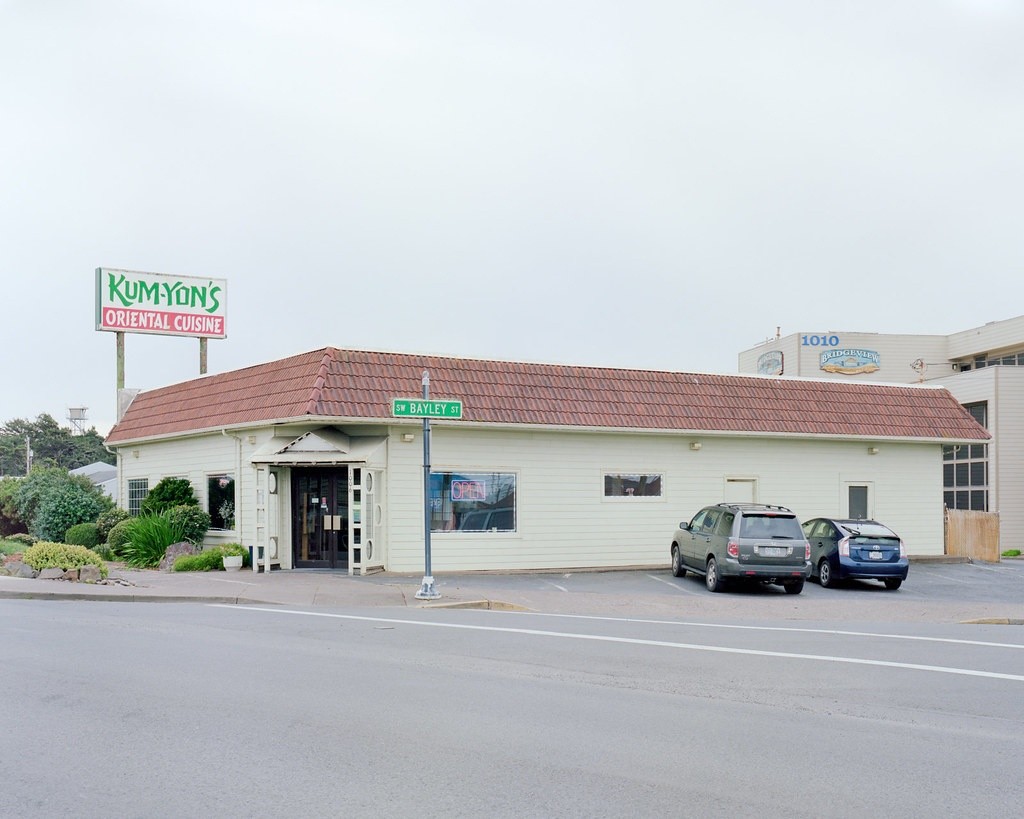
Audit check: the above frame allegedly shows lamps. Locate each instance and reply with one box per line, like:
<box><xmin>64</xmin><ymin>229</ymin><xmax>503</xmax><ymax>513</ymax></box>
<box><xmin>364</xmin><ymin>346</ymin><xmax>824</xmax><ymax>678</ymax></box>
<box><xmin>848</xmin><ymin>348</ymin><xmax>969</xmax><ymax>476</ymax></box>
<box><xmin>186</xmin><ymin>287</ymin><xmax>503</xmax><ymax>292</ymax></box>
<box><xmin>249</xmin><ymin>435</ymin><xmax>256</xmax><ymax>444</ymax></box>
<box><xmin>401</xmin><ymin>433</ymin><xmax>415</xmax><ymax>442</ymax></box>
<box><xmin>132</xmin><ymin>450</ymin><xmax>139</xmax><ymax>459</ymax></box>
<box><xmin>690</xmin><ymin>441</ymin><xmax>702</xmax><ymax>450</ymax></box>
<box><xmin>868</xmin><ymin>448</ymin><xmax>880</xmax><ymax>454</ymax></box>
<box><xmin>219</xmin><ymin>478</ymin><xmax>229</xmax><ymax>488</ymax></box>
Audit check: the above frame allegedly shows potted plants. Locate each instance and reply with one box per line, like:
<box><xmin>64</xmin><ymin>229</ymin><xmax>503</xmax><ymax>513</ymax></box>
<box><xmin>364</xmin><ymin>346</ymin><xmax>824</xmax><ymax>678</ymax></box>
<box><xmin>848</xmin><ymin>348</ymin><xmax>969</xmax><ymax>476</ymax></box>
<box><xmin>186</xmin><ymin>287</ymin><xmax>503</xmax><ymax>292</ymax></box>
<box><xmin>220</xmin><ymin>544</ymin><xmax>243</xmax><ymax>572</ymax></box>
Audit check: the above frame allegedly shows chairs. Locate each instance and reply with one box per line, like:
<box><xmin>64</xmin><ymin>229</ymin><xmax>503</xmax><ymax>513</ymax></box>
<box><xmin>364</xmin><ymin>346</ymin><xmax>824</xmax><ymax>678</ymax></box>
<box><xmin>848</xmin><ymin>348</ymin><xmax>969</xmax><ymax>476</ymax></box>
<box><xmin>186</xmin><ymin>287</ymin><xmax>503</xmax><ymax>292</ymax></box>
<box><xmin>821</xmin><ymin>525</ymin><xmax>834</xmax><ymax>536</ymax></box>
<box><xmin>749</xmin><ymin>519</ymin><xmax>767</xmax><ymax>532</ymax></box>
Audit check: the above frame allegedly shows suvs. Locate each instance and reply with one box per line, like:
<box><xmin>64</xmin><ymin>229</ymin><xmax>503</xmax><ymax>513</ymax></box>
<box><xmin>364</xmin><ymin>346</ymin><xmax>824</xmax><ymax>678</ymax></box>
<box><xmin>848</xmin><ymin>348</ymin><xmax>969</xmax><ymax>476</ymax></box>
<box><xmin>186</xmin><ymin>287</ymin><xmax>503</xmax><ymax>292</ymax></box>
<box><xmin>669</xmin><ymin>502</ymin><xmax>812</xmax><ymax>594</ymax></box>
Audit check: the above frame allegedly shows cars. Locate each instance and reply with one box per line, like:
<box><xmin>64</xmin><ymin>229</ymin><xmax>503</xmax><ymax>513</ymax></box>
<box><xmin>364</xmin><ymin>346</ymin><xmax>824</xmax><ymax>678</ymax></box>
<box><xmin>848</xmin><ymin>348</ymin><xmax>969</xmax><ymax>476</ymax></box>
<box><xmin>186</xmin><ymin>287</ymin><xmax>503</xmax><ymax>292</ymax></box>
<box><xmin>802</xmin><ymin>514</ymin><xmax>909</xmax><ymax>590</ymax></box>
